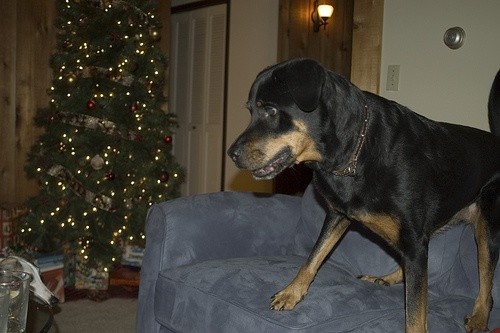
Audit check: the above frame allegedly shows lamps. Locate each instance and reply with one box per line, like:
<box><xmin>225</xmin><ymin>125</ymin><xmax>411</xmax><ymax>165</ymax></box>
<box><xmin>317</xmin><ymin>4</ymin><xmax>334</xmax><ymax>30</ymax></box>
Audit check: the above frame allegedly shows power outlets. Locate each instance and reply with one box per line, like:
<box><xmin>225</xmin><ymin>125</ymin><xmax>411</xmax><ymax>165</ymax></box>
<box><xmin>386</xmin><ymin>65</ymin><xmax>400</xmax><ymax>92</ymax></box>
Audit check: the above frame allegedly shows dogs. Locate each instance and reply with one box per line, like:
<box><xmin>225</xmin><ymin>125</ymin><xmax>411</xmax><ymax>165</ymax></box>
<box><xmin>0</xmin><ymin>255</ymin><xmax>60</xmax><ymax>308</ymax></box>
<box><xmin>226</xmin><ymin>56</ymin><xmax>500</xmax><ymax>333</ymax></box>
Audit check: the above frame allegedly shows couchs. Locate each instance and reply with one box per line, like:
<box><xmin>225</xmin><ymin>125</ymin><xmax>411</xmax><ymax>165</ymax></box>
<box><xmin>136</xmin><ymin>183</ymin><xmax>500</xmax><ymax>333</ymax></box>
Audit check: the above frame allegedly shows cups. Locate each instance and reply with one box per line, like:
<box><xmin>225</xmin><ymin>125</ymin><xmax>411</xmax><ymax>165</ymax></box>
<box><xmin>0</xmin><ymin>257</ymin><xmax>31</xmax><ymax>333</ymax></box>
